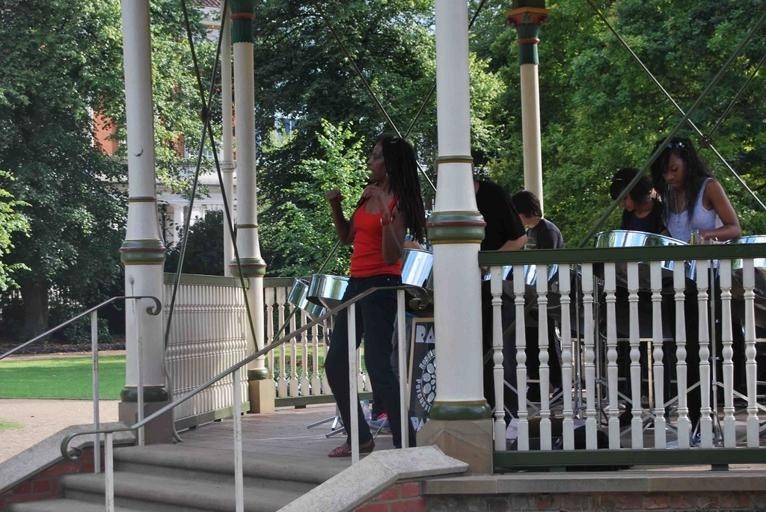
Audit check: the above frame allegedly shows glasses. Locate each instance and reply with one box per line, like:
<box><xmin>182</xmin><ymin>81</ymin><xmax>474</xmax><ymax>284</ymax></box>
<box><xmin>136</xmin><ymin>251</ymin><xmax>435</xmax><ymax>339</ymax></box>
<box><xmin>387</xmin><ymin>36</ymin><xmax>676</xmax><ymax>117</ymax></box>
<box><xmin>653</xmin><ymin>140</ymin><xmax>687</xmax><ymax>154</ymax></box>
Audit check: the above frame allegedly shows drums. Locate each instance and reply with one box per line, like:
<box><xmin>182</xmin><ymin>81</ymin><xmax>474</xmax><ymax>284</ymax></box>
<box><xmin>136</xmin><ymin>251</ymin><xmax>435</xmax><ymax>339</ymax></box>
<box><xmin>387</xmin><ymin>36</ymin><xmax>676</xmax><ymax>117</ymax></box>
<box><xmin>591</xmin><ymin>231</ymin><xmax>697</xmax><ymax>295</ymax></box>
<box><xmin>306</xmin><ymin>272</ymin><xmax>350</xmax><ymax>307</ymax></box>
<box><xmin>400</xmin><ymin>247</ymin><xmax>434</xmax><ymax>303</ymax></box>
<box><xmin>482</xmin><ymin>263</ymin><xmax>574</xmax><ymax>309</ymax></box>
<box><xmin>287</xmin><ymin>277</ymin><xmax>334</xmax><ymax>328</ymax></box>
<box><xmin>715</xmin><ymin>233</ymin><xmax>766</xmax><ymax>297</ymax></box>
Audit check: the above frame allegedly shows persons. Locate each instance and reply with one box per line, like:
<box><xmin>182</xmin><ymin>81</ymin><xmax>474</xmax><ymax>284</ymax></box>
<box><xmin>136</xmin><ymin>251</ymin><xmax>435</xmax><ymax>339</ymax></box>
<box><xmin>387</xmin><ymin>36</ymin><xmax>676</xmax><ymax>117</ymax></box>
<box><xmin>326</xmin><ymin>133</ymin><xmax>428</xmax><ymax>458</ymax></box>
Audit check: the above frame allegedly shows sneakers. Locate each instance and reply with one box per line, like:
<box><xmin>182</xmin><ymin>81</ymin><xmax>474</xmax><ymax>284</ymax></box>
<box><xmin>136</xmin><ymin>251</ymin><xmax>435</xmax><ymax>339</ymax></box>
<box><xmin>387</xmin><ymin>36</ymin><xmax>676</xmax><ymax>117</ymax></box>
<box><xmin>326</xmin><ymin>437</ymin><xmax>375</xmax><ymax>457</ymax></box>
<box><xmin>369</xmin><ymin>411</ymin><xmax>393</xmax><ymax>433</ymax></box>
